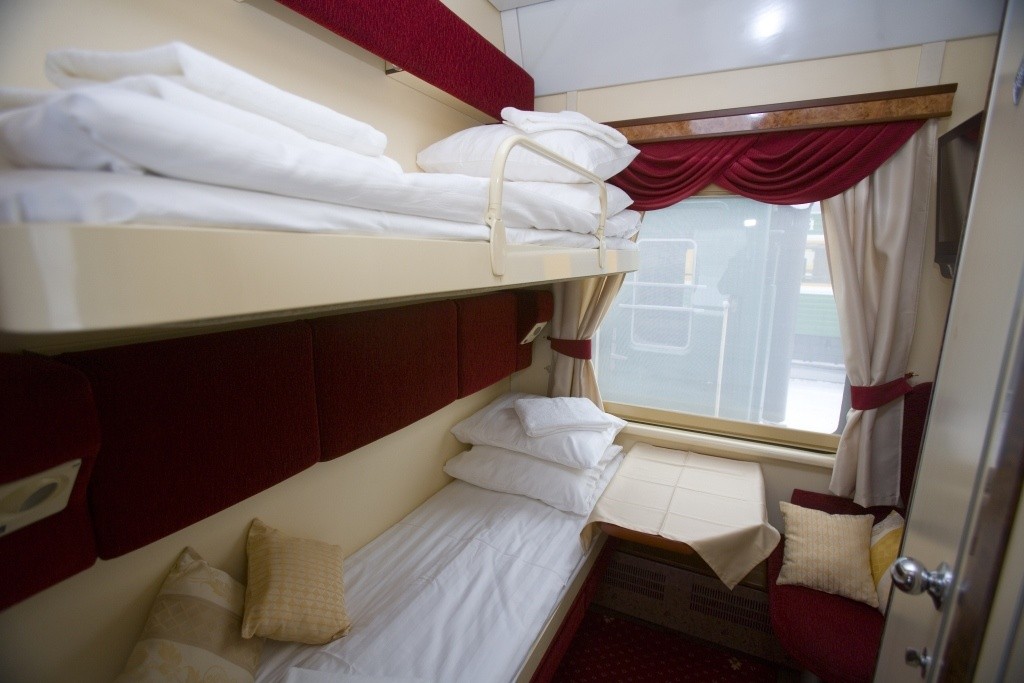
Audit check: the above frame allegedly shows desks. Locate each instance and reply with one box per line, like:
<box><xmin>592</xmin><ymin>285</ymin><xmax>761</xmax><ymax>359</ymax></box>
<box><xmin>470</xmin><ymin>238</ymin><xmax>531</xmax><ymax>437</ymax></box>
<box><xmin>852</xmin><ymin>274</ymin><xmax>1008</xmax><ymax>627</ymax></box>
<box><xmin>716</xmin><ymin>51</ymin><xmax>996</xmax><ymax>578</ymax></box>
<box><xmin>577</xmin><ymin>440</ymin><xmax>782</xmax><ymax>592</ymax></box>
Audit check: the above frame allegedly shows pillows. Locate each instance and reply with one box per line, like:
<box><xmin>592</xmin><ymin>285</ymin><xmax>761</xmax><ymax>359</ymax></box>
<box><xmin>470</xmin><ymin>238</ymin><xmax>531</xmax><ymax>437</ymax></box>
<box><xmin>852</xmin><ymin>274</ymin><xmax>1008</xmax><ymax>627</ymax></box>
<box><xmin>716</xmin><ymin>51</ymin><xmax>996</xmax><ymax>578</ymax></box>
<box><xmin>240</xmin><ymin>516</ymin><xmax>352</xmax><ymax>645</ymax></box>
<box><xmin>114</xmin><ymin>546</ymin><xmax>269</xmax><ymax>683</ymax></box>
<box><xmin>507</xmin><ymin>180</ymin><xmax>637</xmax><ymax>219</ymax></box>
<box><xmin>416</xmin><ymin>124</ymin><xmax>640</xmax><ymax>183</ymax></box>
<box><xmin>775</xmin><ymin>500</ymin><xmax>881</xmax><ymax>608</ymax></box>
<box><xmin>870</xmin><ymin>509</ymin><xmax>907</xmax><ymax>617</ymax></box>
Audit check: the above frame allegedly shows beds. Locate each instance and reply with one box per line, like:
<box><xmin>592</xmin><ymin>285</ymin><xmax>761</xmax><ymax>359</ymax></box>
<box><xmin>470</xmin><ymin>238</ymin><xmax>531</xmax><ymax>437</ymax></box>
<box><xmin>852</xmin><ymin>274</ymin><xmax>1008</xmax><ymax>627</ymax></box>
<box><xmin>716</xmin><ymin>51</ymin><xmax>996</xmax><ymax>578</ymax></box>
<box><xmin>3</xmin><ymin>86</ymin><xmax>642</xmax><ymax>336</ymax></box>
<box><xmin>237</xmin><ymin>442</ymin><xmax>630</xmax><ymax>683</ymax></box>
